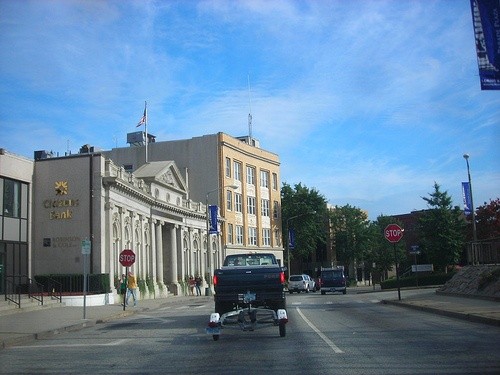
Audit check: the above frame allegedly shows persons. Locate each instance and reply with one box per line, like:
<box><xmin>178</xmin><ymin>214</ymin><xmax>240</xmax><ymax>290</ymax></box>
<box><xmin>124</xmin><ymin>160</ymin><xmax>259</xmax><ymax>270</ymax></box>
<box><xmin>188</xmin><ymin>274</ymin><xmax>203</xmax><ymax>296</ymax></box>
<box><xmin>116</xmin><ymin>272</ymin><xmax>137</xmax><ymax>307</ymax></box>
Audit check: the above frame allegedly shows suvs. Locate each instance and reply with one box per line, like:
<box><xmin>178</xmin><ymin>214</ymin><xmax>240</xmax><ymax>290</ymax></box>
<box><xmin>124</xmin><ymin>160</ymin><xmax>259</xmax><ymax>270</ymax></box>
<box><xmin>288</xmin><ymin>274</ymin><xmax>317</xmax><ymax>294</ymax></box>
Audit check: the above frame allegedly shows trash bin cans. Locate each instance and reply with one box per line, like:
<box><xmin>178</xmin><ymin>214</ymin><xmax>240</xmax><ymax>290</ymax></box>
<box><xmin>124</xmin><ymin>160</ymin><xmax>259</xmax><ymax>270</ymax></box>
<box><xmin>205</xmin><ymin>288</ymin><xmax>209</xmax><ymax>296</ymax></box>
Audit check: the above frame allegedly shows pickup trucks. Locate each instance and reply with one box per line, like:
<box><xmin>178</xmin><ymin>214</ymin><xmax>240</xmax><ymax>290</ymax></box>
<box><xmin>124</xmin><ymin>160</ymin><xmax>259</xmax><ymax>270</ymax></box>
<box><xmin>213</xmin><ymin>253</ymin><xmax>287</xmax><ymax>319</ymax></box>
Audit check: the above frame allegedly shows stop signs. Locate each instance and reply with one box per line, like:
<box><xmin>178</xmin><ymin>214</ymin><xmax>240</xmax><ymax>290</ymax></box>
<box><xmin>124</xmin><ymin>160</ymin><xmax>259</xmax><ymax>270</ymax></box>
<box><xmin>384</xmin><ymin>224</ymin><xmax>403</xmax><ymax>242</ymax></box>
<box><xmin>119</xmin><ymin>249</ymin><xmax>135</xmax><ymax>266</ymax></box>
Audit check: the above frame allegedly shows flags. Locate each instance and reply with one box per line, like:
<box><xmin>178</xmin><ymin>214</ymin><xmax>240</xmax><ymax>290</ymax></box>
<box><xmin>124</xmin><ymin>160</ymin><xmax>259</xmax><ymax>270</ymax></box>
<box><xmin>136</xmin><ymin>110</ymin><xmax>146</xmax><ymax>128</ymax></box>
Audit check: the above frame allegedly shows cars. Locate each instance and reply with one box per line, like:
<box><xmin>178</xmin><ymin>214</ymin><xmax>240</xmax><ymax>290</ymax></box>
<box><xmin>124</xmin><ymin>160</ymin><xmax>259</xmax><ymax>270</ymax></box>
<box><xmin>318</xmin><ymin>265</ymin><xmax>349</xmax><ymax>295</ymax></box>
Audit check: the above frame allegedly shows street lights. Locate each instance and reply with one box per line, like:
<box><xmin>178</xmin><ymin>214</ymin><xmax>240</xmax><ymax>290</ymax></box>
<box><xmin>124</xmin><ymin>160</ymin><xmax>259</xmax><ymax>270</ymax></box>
<box><xmin>286</xmin><ymin>210</ymin><xmax>316</xmax><ymax>279</ymax></box>
<box><xmin>206</xmin><ymin>185</ymin><xmax>238</xmax><ymax>296</ymax></box>
<box><xmin>463</xmin><ymin>154</ymin><xmax>479</xmax><ymax>266</ymax></box>
